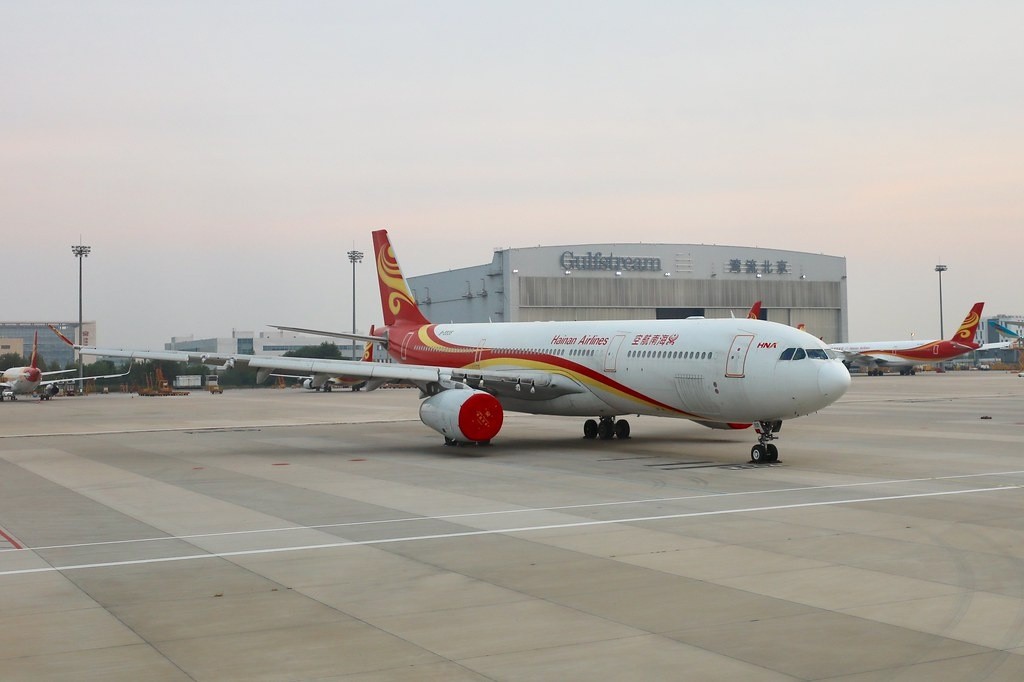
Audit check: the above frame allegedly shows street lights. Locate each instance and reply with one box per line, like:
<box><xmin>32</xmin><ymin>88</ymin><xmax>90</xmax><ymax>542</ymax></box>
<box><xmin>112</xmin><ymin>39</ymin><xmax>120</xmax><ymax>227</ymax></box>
<box><xmin>934</xmin><ymin>264</ymin><xmax>948</xmax><ymax>372</ymax></box>
<box><xmin>70</xmin><ymin>245</ymin><xmax>91</xmax><ymax>395</ymax></box>
<box><xmin>345</xmin><ymin>250</ymin><xmax>365</xmax><ymax>360</ymax></box>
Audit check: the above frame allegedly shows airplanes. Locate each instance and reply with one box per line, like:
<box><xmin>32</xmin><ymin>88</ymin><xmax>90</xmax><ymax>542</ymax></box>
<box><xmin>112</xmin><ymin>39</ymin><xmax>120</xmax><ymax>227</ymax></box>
<box><xmin>47</xmin><ymin>229</ymin><xmax>850</xmax><ymax>466</ymax></box>
<box><xmin>797</xmin><ymin>301</ymin><xmax>986</xmax><ymax>375</ymax></box>
<box><xmin>0</xmin><ymin>328</ymin><xmax>131</xmax><ymax>402</ymax></box>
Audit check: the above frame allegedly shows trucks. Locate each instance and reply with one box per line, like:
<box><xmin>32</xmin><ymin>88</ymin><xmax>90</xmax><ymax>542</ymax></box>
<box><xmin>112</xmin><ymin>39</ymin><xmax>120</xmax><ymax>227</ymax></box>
<box><xmin>173</xmin><ymin>374</ymin><xmax>219</xmax><ymax>389</ymax></box>
